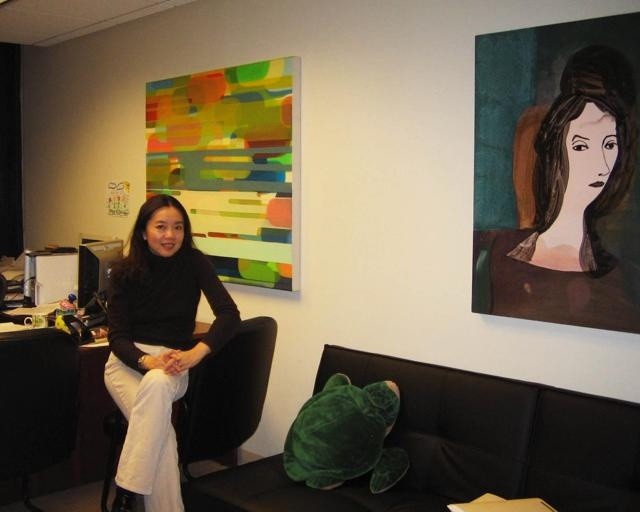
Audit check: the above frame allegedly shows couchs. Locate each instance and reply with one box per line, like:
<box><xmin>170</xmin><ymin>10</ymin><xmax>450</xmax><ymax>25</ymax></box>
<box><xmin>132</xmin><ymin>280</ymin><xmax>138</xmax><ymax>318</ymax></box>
<box><xmin>181</xmin><ymin>345</ymin><xmax>640</xmax><ymax>512</ymax></box>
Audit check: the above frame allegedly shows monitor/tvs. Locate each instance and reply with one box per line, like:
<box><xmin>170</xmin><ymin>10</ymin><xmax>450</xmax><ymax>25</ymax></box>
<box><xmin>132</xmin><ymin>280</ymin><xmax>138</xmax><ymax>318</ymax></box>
<box><xmin>78</xmin><ymin>239</ymin><xmax>124</xmax><ymax>327</ymax></box>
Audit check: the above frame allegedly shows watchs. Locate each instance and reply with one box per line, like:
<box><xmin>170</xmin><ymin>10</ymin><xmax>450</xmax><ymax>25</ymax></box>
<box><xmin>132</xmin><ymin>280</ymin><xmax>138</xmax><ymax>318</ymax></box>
<box><xmin>136</xmin><ymin>354</ymin><xmax>146</xmax><ymax>369</ymax></box>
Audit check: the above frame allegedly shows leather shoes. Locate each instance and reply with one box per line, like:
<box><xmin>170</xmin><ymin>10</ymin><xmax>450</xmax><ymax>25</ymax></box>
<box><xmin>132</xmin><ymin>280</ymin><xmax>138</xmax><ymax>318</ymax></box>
<box><xmin>112</xmin><ymin>487</ymin><xmax>137</xmax><ymax>512</ymax></box>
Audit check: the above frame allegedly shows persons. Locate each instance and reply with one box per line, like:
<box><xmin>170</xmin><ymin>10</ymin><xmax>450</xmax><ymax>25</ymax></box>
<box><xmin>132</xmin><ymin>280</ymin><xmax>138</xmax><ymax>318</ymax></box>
<box><xmin>103</xmin><ymin>194</ymin><xmax>241</xmax><ymax>511</ymax></box>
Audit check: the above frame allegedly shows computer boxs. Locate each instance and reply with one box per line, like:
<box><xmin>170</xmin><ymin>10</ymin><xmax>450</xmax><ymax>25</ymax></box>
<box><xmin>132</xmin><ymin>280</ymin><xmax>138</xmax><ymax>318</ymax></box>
<box><xmin>23</xmin><ymin>246</ymin><xmax>79</xmax><ymax>307</ymax></box>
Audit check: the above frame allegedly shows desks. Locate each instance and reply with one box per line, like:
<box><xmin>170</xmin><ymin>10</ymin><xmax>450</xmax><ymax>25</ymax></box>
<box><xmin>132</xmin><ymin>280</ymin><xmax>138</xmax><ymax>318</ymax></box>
<box><xmin>0</xmin><ymin>322</ymin><xmax>212</xmax><ymax>503</ymax></box>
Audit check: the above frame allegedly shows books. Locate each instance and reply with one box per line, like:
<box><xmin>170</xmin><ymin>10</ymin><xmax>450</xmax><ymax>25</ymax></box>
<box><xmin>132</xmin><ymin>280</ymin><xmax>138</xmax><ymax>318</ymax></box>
<box><xmin>446</xmin><ymin>496</ymin><xmax>557</xmax><ymax>511</ymax></box>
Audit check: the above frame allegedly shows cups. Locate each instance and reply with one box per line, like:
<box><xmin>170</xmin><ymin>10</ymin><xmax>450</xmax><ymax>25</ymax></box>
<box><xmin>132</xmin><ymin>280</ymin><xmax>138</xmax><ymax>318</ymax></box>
<box><xmin>24</xmin><ymin>313</ymin><xmax>49</xmax><ymax>330</ymax></box>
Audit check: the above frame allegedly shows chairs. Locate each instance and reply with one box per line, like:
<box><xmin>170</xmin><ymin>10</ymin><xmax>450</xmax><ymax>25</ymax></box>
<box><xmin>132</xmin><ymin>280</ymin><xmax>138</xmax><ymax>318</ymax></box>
<box><xmin>102</xmin><ymin>316</ymin><xmax>277</xmax><ymax>512</ymax></box>
<box><xmin>0</xmin><ymin>328</ymin><xmax>79</xmax><ymax>512</ymax></box>
